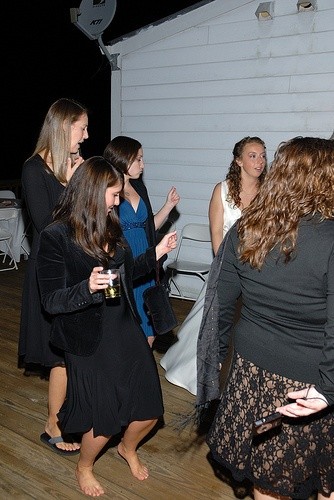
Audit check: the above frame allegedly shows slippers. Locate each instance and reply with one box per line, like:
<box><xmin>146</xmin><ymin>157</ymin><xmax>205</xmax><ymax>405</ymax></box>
<box><xmin>39</xmin><ymin>431</ymin><xmax>81</xmax><ymax>457</ymax></box>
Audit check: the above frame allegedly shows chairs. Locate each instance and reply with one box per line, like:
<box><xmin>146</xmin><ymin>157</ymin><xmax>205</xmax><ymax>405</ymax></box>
<box><xmin>0</xmin><ymin>190</ymin><xmax>16</xmax><ymax>200</ymax></box>
<box><xmin>166</xmin><ymin>221</ymin><xmax>212</xmax><ymax>301</ymax></box>
<box><xmin>0</xmin><ymin>208</ymin><xmax>21</xmax><ymax>271</ymax></box>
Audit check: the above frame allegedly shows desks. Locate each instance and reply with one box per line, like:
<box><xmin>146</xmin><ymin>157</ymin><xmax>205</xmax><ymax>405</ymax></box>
<box><xmin>0</xmin><ymin>198</ymin><xmax>31</xmax><ymax>262</ymax></box>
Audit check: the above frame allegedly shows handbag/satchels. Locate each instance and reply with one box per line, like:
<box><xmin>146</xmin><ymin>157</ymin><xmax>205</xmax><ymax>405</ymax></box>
<box><xmin>0</xmin><ymin>198</ymin><xmax>22</xmax><ymax>209</ymax></box>
<box><xmin>144</xmin><ymin>260</ymin><xmax>178</xmax><ymax>335</ymax></box>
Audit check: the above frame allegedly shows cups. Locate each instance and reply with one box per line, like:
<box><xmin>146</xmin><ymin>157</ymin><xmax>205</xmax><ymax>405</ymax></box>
<box><xmin>99</xmin><ymin>269</ymin><xmax>121</xmax><ymax>299</ymax></box>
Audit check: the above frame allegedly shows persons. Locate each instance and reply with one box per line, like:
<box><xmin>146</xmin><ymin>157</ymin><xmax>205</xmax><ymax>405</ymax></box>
<box><xmin>160</xmin><ymin>137</ymin><xmax>269</xmax><ymax>396</ymax></box>
<box><xmin>15</xmin><ymin>98</ymin><xmax>89</xmax><ymax>454</ymax></box>
<box><xmin>33</xmin><ymin>154</ymin><xmax>164</xmax><ymax>497</ymax></box>
<box><xmin>195</xmin><ymin>137</ymin><xmax>334</xmax><ymax>500</ymax></box>
<box><xmin>104</xmin><ymin>136</ymin><xmax>180</xmax><ymax>347</ymax></box>
